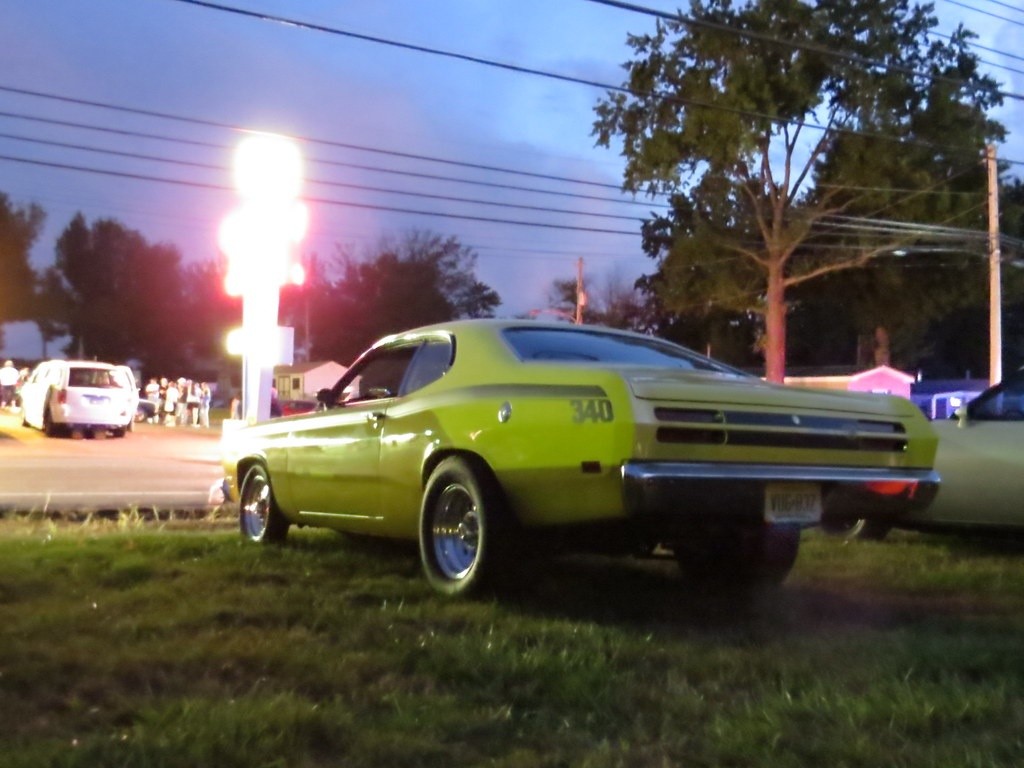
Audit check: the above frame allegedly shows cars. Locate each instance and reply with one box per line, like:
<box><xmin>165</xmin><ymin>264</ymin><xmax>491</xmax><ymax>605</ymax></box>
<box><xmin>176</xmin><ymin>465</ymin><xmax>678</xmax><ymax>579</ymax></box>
<box><xmin>216</xmin><ymin>318</ymin><xmax>941</xmax><ymax>596</ymax></box>
<box><xmin>815</xmin><ymin>367</ymin><xmax>1024</xmax><ymax>542</ymax></box>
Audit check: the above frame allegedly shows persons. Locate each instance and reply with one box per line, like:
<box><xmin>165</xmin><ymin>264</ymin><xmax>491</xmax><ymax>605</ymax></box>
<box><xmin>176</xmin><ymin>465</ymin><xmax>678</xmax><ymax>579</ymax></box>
<box><xmin>0</xmin><ymin>360</ymin><xmax>30</xmax><ymax>407</ymax></box>
<box><xmin>271</xmin><ymin>387</ymin><xmax>283</xmax><ymax>418</ymax></box>
<box><xmin>230</xmin><ymin>391</ymin><xmax>241</xmax><ymax>419</ymax></box>
<box><xmin>144</xmin><ymin>377</ymin><xmax>211</xmax><ymax>427</ymax></box>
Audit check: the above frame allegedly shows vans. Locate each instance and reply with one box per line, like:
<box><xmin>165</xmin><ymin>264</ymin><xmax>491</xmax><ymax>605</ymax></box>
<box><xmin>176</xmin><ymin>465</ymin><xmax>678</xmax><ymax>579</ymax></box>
<box><xmin>19</xmin><ymin>360</ymin><xmax>159</xmax><ymax>439</ymax></box>
